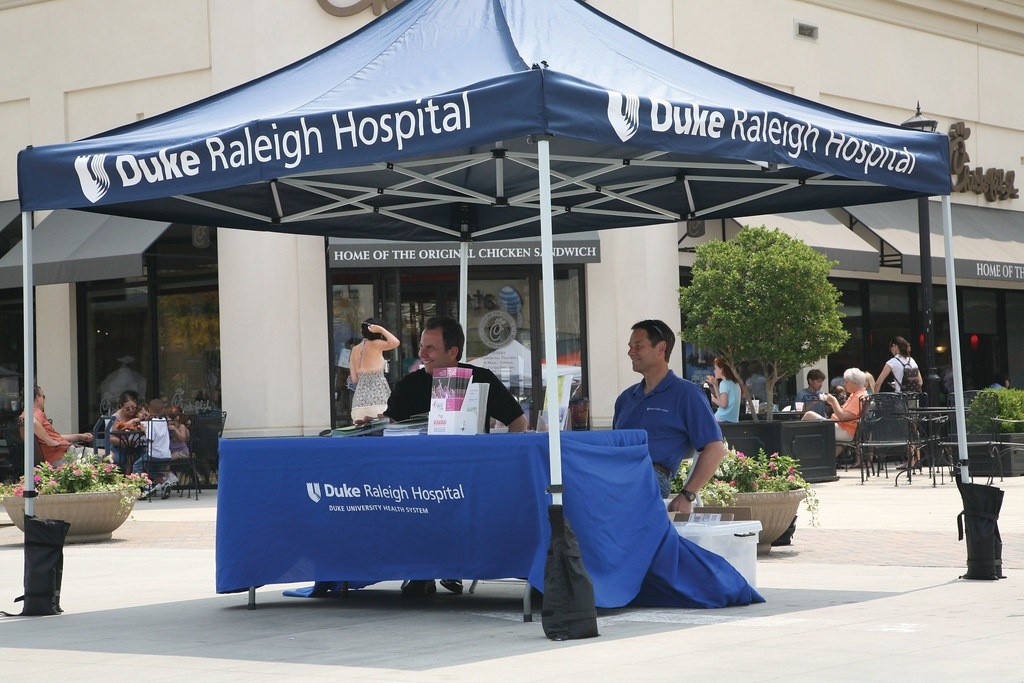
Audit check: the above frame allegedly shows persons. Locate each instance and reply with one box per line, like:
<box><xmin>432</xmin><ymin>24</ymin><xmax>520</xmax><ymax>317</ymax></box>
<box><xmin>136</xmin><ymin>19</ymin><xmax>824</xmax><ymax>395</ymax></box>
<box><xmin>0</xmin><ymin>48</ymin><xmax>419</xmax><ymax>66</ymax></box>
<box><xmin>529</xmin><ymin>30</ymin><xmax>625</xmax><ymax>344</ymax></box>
<box><xmin>350</xmin><ymin>318</ymin><xmax>400</xmax><ymax>426</ymax></box>
<box><xmin>19</xmin><ymin>387</ymin><xmax>115</xmax><ymax>469</ymax></box>
<box><xmin>874</xmin><ymin>336</ymin><xmax>923</xmax><ymax>470</ymax></box>
<box><xmin>335</xmin><ymin>337</ymin><xmax>361</xmax><ymax>427</ymax></box>
<box><xmin>706</xmin><ymin>355</ymin><xmax>741</xmax><ymax>423</ymax></box>
<box><xmin>109</xmin><ymin>390</ymin><xmax>218</xmax><ymax>500</ymax></box>
<box><xmin>355</xmin><ymin>316</ymin><xmax>528</xmax><ymax>596</ymax></box>
<box><xmin>743</xmin><ymin>361</ymin><xmax>772</xmax><ymax>399</ymax></box>
<box><xmin>793</xmin><ymin>368</ymin><xmax>875</xmax><ymax>469</ymax></box>
<box><xmin>612</xmin><ymin>320</ymin><xmax>725</xmax><ymax>514</ymax></box>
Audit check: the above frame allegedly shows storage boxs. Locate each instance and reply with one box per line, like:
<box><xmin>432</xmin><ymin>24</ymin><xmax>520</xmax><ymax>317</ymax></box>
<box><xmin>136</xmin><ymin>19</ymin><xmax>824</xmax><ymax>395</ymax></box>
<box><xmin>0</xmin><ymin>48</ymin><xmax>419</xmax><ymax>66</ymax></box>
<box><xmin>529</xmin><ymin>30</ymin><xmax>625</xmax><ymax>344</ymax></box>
<box><xmin>667</xmin><ymin>518</ymin><xmax>762</xmax><ymax>589</ymax></box>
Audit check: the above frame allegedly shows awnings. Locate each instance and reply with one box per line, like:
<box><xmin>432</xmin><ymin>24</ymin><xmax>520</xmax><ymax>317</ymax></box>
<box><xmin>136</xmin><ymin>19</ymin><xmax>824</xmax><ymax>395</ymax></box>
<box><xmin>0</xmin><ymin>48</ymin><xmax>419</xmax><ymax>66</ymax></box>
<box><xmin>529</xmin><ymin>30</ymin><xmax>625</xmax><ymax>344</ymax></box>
<box><xmin>0</xmin><ymin>200</ymin><xmax>172</xmax><ymax>288</ymax></box>
<box><xmin>732</xmin><ymin>209</ymin><xmax>880</xmax><ymax>273</ymax></box>
<box><xmin>841</xmin><ymin>198</ymin><xmax>1024</xmax><ymax>283</ymax></box>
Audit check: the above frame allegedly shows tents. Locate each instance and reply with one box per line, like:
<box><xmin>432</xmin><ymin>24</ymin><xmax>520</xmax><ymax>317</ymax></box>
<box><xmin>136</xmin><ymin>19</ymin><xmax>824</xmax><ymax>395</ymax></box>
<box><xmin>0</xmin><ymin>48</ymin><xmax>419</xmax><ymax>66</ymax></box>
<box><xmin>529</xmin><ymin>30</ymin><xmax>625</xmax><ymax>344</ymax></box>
<box><xmin>18</xmin><ymin>0</ymin><xmax>1004</xmax><ymax>622</ymax></box>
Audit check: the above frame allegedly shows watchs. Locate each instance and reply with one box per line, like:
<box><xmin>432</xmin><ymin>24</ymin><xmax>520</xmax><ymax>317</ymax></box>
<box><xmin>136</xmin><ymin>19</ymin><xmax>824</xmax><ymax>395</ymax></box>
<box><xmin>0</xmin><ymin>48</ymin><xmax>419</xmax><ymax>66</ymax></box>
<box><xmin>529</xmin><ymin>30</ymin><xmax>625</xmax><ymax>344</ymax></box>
<box><xmin>680</xmin><ymin>489</ymin><xmax>696</xmax><ymax>502</ymax></box>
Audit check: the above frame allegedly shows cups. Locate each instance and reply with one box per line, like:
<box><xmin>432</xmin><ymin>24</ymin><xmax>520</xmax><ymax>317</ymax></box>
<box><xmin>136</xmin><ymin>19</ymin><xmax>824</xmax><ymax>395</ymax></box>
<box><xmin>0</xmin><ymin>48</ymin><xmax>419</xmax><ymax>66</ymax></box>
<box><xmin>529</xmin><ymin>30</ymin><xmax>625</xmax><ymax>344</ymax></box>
<box><xmin>384</xmin><ymin>360</ymin><xmax>389</xmax><ymax>373</ymax></box>
<box><xmin>10</xmin><ymin>401</ymin><xmax>17</xmax><ymax>411</ymax></box>
<box><xmin>752</xmin><ymin>400</ymin><xmax>759</xmax><ymax>414</ymax></box>
<box><xmin>796</xmin><ymin>402</ymin><xmax>804</xmax><ymax>412</ymax></box>
<box><xmin>104</xmin><ymin>419</ymin><xmax>113</xmax><ymax>432</ymax></box>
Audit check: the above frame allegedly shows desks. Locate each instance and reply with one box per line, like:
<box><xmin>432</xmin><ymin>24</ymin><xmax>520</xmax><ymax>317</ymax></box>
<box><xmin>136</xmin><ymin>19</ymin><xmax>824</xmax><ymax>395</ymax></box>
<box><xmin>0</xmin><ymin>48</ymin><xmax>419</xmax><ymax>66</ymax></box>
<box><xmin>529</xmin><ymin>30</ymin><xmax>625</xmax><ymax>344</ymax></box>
<box><xmin>90</xmin><ymin>426</ymin><xmax>172</xmax><ymax>476</ymax></box>
<box><xmin>214</xmin><ymin>430</ymin><xmax>766</xmax><ymax>622</ymax></box>
<box><xmin>759</xmin><ymin>411</ymin><xmax>806</xmax><ymax>421</ymax></box>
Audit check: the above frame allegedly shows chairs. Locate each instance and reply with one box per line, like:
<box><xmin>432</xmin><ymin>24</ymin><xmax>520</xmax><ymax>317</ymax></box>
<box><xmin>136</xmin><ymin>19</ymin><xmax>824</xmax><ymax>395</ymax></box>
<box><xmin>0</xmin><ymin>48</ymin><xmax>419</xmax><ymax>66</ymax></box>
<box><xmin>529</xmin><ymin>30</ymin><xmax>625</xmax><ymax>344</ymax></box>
<box><xmin>799</xmin><ymin>390</ymin><xmax>1023</xmax><ymax>488</ymax></box>
<box><xmin>662</xmin><ymin>444</ymin><xmax>705</xmax><ymax>509</ymax></box>
<box><xmin>2</xmin><ymin>409</ymin><xmax>227</xmax><ymax>500</ymax></box>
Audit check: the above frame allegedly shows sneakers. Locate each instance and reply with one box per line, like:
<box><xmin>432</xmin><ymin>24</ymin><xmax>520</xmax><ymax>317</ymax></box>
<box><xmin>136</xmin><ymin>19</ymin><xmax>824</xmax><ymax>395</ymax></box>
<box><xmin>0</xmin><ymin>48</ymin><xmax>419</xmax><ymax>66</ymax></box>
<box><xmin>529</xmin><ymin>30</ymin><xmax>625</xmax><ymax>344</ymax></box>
<box><xmin>161</xmin><ymin>481</ymin><xmax>171</xmax><ymax>499</ymax></box>
<box><xmin>138</xmin><ymin>481</ymin><xmax>160</xmax><ymax>501</ymax></box>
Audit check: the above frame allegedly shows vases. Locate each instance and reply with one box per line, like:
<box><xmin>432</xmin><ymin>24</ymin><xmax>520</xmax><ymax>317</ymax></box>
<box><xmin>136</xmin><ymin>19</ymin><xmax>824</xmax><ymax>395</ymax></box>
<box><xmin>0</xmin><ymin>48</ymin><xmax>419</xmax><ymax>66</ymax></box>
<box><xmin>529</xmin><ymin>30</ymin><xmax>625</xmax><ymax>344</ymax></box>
<box><xmin>3</xmin><ymin>486</ymin><xmax>141</xmax><ymax>543</ymax></box>
<box><xmin>706</xmin><ymin>488</ymin><xmax>806</xmax><ymax>555</ymax></box>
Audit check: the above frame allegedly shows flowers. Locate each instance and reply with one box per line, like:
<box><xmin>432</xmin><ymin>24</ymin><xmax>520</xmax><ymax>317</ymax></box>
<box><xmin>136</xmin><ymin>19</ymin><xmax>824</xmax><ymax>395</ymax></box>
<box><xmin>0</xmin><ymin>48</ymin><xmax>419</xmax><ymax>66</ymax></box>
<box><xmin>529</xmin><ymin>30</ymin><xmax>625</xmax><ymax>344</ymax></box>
<box><xmin>0</xmin><ymin>454</ymin><xmax>153</xmax><ymax>522</ymax></box>
<box><xmin>659</xmin><ymin>442</ymin><xmax>820</xmax><ymax>530</ymax></box>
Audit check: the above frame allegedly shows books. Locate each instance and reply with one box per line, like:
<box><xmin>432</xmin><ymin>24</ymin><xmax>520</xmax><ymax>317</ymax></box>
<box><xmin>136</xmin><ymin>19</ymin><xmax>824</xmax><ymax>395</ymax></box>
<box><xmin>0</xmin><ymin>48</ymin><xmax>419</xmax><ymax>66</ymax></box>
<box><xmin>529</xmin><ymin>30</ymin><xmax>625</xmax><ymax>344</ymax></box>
<box><xmin>427</xmin><ymin>367</ymin><xmax>490</xmax><ymax>435</ymax></box>
<box><xmin>332</xmin><ymin>412</ymin><xmax>428</xmax><ymax>437</ymax></box>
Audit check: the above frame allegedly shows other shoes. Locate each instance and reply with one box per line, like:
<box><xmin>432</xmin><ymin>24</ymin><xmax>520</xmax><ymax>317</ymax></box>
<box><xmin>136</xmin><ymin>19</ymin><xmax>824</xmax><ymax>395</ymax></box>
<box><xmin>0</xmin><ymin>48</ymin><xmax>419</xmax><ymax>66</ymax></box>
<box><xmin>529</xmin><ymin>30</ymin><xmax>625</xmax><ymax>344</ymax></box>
<box><xmin>440</xmin><ymin>579</ymin><xmax>462</xmax><ymax>593</ymax></box>
<box><xmin>405</xmin><ymin>581</ymin><xmax>435</xmax><ymax>593</ymax></box>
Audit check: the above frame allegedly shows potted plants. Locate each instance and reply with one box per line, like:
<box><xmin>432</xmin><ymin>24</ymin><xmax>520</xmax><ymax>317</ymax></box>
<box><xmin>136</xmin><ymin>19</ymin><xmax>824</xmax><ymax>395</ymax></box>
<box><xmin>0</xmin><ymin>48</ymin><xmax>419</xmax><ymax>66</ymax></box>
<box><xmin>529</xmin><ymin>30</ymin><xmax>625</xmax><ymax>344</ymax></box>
<box><xmin>944</xmin><ymin>387</ymin><xmax>1023</xmax><ymax>478</ymax></box>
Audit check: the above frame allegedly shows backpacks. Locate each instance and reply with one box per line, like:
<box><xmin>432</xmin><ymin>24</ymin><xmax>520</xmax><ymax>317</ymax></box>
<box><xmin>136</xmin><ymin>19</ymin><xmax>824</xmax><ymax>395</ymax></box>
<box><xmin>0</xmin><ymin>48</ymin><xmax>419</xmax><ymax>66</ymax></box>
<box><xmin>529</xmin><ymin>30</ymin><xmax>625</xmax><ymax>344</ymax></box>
<box><xmin>893</xmin><ymin>356</ymin><xmax>920</xmax><ymax>392</ymax></box>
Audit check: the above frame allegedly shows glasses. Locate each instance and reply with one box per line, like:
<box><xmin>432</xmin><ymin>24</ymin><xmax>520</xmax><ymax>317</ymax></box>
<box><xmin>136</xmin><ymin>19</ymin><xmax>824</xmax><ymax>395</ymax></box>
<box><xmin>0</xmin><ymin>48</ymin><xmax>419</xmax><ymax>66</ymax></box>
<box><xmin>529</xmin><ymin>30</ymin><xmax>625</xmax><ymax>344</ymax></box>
<box><xmin>121</xmin><ymin>402</ymin><xmax>138</xmax><ymax>411</ymax></box>
<box><xmin>40</xmin><ymin>395</ymin><xmax>45</xmax><ymax>398</ymax></box>
